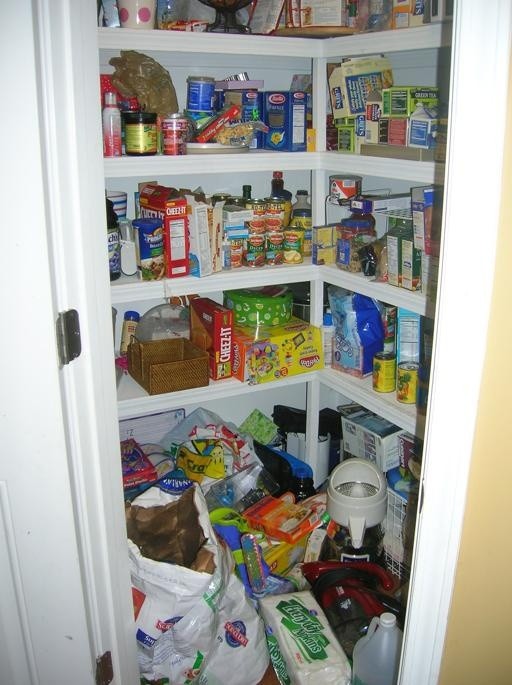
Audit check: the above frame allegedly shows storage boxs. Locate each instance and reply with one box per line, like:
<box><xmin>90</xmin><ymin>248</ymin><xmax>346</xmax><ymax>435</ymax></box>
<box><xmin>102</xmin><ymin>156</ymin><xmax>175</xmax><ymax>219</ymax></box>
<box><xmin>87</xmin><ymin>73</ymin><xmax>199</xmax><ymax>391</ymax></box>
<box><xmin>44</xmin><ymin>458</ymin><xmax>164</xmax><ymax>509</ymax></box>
<box><xmin>230</xmin><ymin>317</ymin><xmax>325</xmax><ymax>386</ymax></box>
<box><xmin>341</xmin><ymin>409</ymin><xmax>406</xmax><ymax>472</ymax></box>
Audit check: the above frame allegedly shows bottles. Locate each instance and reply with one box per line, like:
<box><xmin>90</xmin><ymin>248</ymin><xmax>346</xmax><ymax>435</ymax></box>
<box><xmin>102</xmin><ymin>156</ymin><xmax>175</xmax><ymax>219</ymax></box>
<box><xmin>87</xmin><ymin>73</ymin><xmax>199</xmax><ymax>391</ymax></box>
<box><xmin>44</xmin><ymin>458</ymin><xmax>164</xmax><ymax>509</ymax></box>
<box><xmin>241</xmin><ymin>171</ymin><xmax>312</xmax><ymax>216</ymax></box>
<box><xmin>103</xmin><ymin>91</ymin><xmax>122</xmax><ymax>157</ymax></box>
<box><xmin>336</xmin><ymin>198</ymin><xmax>376</xmax><ymax>273</ymax></box>
<box><xmin>292</xmin><ymin>467</ymin><xmax>315</xmax><ymax>503</ymax></box>
<box><xmin>104</xmin><ymin>187</ymin><xmax>120</xmax><ymax>281</ymax></box>
<box><xmin>120</xmin><ymin>310</ymin><xmax>140</xmax><ymax>357</ymax></box>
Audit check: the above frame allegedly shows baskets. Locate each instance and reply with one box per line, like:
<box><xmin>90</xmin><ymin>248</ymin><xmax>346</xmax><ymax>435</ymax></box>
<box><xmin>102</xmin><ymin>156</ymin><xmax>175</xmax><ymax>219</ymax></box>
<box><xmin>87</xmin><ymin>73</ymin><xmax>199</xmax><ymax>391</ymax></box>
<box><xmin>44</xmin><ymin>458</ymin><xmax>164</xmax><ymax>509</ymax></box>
<box><xmin>383</xmin><ymin>487</ymin><xmax>409</xmax><ymax>581</ymax></box>
<box><xmin>127</xmin><ymin>335</ymin><xmax>210</xmax><ymax>396</ymax></box>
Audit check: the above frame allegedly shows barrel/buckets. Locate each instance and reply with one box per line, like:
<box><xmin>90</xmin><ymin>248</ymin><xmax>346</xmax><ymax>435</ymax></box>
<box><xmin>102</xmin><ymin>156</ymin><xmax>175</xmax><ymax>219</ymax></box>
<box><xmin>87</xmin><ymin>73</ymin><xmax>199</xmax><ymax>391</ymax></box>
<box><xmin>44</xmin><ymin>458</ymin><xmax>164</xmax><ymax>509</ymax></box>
<box><xmin>352</xmin><ymin>612</ymin><xmax>403</xmax><ymax>685</ymax></box>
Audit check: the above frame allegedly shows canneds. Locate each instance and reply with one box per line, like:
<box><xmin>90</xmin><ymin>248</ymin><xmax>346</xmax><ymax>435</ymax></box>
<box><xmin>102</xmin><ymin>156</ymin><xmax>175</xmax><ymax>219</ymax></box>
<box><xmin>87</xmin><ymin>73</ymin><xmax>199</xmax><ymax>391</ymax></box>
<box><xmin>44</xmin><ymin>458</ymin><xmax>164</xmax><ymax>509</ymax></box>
<box><xmin>224</xmin><ymin>198</ymin><xmax>305</xmax><ymax>268</ymax></box>
<box><xmin>396</xmin><ymin>360</ymin><xmax>420</xmax><ymax>403</ymax></box>
<box><xmin>163</xmin><ymin>117</ymin><xmax>188</xmax><ymax>155</ymax></box>
<box><xmin>373</xmin><ymin>352</ymin><xmax>396</xmax><ymax>393</ymax></box>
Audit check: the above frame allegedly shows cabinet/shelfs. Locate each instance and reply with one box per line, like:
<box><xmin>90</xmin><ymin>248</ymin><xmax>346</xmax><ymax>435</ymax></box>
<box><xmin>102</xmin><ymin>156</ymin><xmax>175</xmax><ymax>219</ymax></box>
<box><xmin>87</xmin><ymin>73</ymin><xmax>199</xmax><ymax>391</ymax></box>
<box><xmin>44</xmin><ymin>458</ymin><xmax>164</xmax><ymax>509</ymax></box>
<box><xmin>96</xmin><ymin>0</ymin><xmax>455</xmax><ymax>489</ymax></box>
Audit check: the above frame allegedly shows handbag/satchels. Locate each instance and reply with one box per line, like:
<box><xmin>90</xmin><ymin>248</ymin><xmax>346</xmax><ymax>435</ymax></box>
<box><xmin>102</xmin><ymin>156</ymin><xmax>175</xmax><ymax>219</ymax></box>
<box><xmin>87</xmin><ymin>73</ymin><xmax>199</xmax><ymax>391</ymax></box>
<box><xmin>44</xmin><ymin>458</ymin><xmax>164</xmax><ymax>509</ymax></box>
<box><xmin>128</xmin><ymin>484</ymin><xmax>271</xmax><ymax>685</ymax></box>
